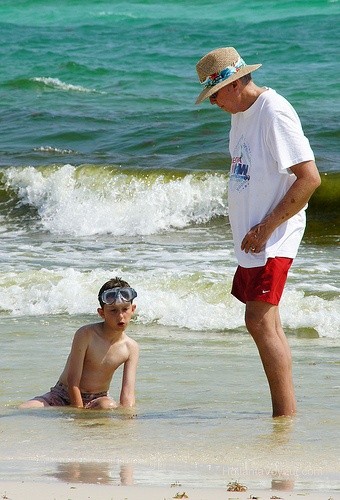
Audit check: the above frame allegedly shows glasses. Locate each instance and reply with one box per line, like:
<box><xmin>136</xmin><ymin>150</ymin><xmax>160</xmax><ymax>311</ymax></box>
<box><xmin>210</xmin><ymin>92</ymin><xmax>219</xmax><ymax>98</ymax></box>
<box><xmin>99</xmin><ymin>287</ymin><xmax>137</xmax><ymax>307</ymax></box>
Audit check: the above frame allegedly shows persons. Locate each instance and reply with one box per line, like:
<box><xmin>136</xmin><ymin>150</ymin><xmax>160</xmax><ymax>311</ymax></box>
<box><xmin>195</xmin><ymin>46</ymin><xmax>322</xmax><ymax>417</ymax></box>
<box><xmin>17</xmin><ymin>276</ymin><xmax>141</xmax><ymax>409</ymax></box>
<box><xmin>56</xmin><ymin>462</ymin><xmax>137</xmax><ymax>485</ymax></box>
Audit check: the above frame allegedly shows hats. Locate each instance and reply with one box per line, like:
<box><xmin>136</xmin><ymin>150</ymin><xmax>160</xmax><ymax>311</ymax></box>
<box><xmin>195</xmin><ymin>47</ymin><xmax>263</xmax><ymax>105</ymax></box>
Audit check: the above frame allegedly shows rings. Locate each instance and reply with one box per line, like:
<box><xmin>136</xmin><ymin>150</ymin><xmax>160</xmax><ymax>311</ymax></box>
<box><xmin>251</xmin><ymin>248</ymin><xmax>255</xmax><ymax>251</ymax></box>
